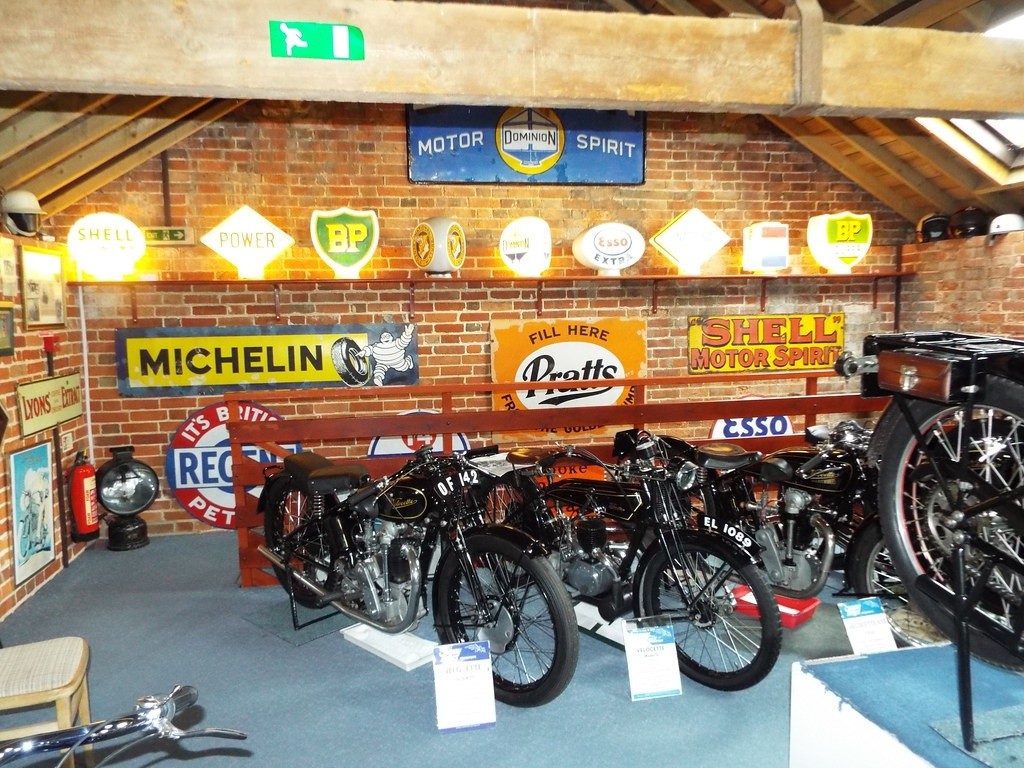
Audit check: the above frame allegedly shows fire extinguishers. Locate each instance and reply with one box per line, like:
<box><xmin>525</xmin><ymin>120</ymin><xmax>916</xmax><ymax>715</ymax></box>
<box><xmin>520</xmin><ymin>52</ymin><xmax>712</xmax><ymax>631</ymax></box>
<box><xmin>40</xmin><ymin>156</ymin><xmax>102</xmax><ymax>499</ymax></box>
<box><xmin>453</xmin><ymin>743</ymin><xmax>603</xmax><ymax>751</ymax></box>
<box><xmin>65</xmin><ymin>449</ymin><xmax>101</xmax><ymax>544</ymax></box>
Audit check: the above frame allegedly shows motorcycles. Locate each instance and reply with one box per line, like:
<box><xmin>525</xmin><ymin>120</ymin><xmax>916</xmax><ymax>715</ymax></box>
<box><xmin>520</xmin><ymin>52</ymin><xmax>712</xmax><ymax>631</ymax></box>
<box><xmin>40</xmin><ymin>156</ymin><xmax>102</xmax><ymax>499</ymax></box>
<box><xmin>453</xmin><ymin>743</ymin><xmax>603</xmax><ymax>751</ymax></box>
<box><xmin>459</xmin><ymin>430</ymin><xmax>784</xmax><ymax>692</ymax></box>
<box><xmin>655</xmin><ymin>423</ymin><xmax>973</xmax><ymax>652</ymax></box>
<box><xmin>254</xmin><ymin>444</ymin><xmax>580</xmax><ymax>708</ymax></box>
<box><xmin>833</xmin><ymin>328</ymin><xmax>1024</xmax><ymax>676</ymax></box>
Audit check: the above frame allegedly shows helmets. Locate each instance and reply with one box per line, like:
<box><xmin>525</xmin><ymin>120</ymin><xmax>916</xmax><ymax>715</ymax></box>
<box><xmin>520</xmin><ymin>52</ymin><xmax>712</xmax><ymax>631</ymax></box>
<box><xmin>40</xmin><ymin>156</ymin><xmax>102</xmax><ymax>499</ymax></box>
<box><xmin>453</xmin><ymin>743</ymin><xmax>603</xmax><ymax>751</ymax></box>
<box><xmin>916</xmin><ymin>212</ymin><xmax>950</xmax><ymax>243</ymax></box>
<box><xmin>947</xmin><ymin>208</ymin><xmax>988</xmax><ymax>238</ymax></box>
<box><xmin>988</xmin><ymin>213</ymin><xmax>1024</xmax><ymax>235</ymax></box>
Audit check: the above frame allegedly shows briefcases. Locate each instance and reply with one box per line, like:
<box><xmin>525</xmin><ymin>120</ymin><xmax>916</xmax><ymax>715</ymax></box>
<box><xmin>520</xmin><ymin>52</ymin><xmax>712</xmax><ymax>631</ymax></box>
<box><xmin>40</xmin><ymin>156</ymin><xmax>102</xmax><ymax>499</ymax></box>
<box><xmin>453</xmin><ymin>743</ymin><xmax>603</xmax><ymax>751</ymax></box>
<box><xmin>877</xmin><ymin>335</ymin><xmax>1024</xmax><ymax>406</ymax></box>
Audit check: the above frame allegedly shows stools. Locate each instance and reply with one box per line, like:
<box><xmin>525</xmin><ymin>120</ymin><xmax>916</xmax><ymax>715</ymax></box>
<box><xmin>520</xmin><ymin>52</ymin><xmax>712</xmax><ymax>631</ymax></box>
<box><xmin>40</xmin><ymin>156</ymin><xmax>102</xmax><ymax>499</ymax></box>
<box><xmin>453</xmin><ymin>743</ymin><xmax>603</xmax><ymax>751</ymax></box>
<box><xmin>0</xmin><ymin>637</ymin><xmax>94</xmax><ymax>768</ymax></box>
